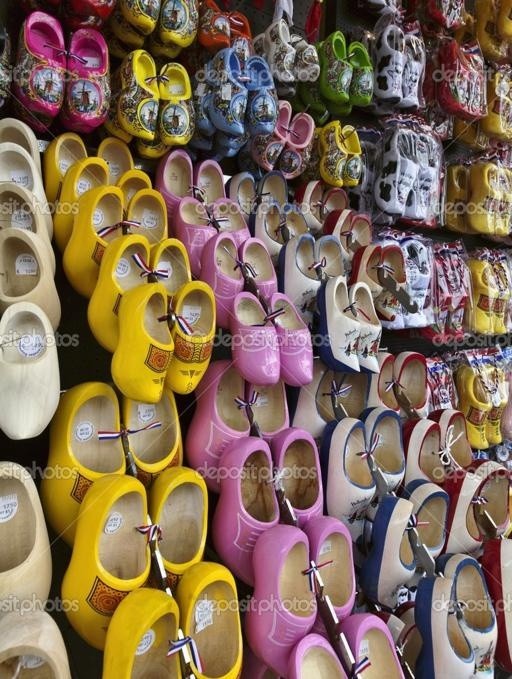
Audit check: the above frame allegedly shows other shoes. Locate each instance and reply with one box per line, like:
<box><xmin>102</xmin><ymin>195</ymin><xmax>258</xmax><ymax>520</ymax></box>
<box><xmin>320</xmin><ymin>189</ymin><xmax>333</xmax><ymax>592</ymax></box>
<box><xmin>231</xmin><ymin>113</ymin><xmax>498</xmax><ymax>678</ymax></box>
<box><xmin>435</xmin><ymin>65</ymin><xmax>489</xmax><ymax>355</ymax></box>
<box><xmin>0</xmin><ymin>0</ymin><xmax>512</xmax><ymax>679</ymax></box>
